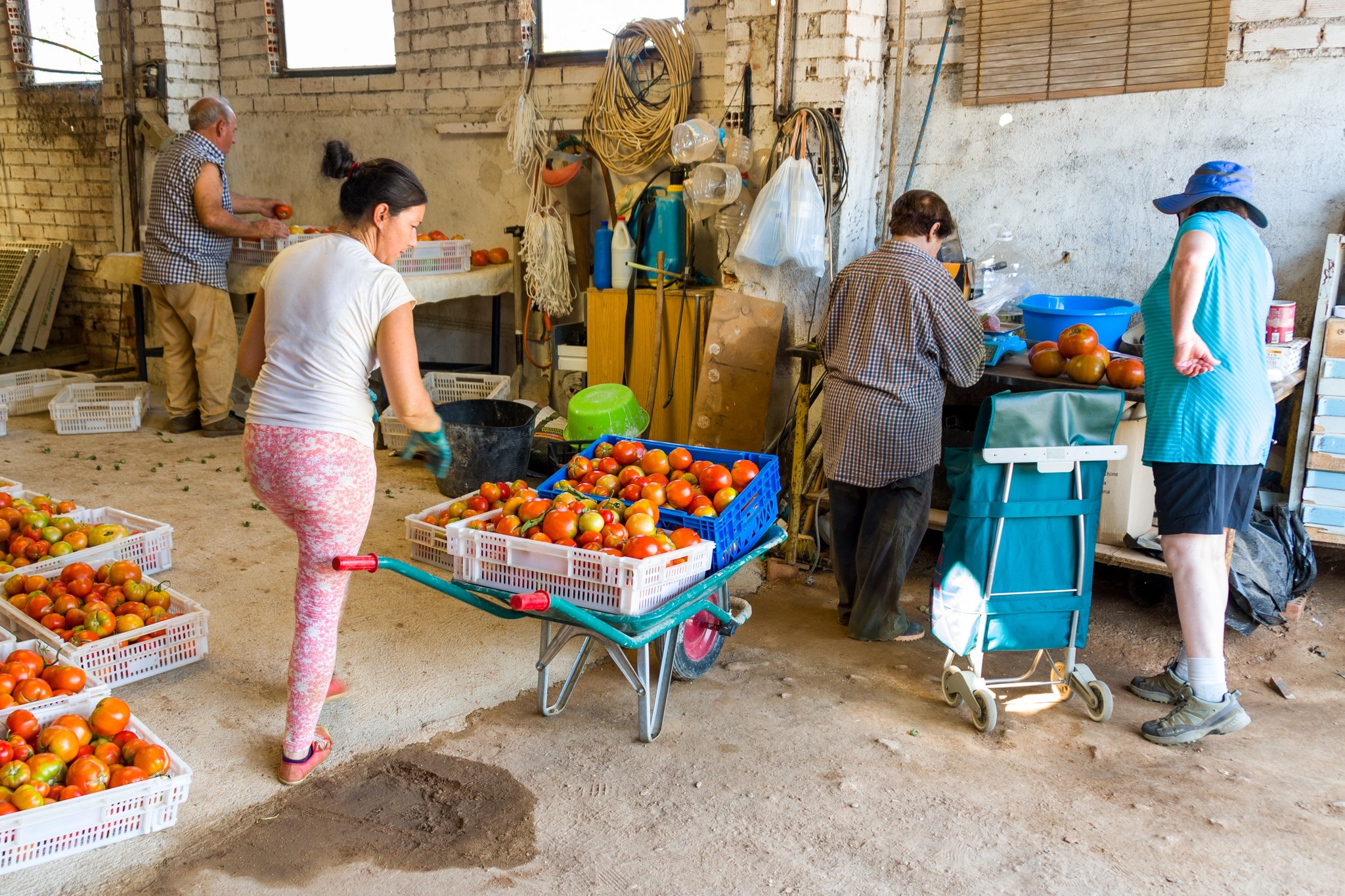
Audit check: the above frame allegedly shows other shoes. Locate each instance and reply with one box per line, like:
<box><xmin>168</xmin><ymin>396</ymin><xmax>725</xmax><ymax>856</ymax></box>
<box><xmin>889</xmin><ymin>622</ymin><xmax>925</xmax><ymax>641</ymax></box>
<box><xmin>169</xmin><ymin>408</ymin><xmax>203</xmax><ymax>432</ymax></box>
<box><xmin>200</xmin><ymin>414</ymin><xmax>246</xmax><ymax>437</ymax></box>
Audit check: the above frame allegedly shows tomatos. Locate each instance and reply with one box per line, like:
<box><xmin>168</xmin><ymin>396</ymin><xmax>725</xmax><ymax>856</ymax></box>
<box><xmin>0</xmin><ymin>640</ymin><xmax>177</xmax><ymax>860</ymax></box>
<box><xmin>1089</xmin><ymin>343</ymin><xmax>1110</xmax><ymax>369</ymax></box>
<box><xmin>1031</xmin><ymin>348</ymin><xmax>1067</xmax><ymax>377</ymax></box>
<box><xmin>552</xmin><ymin>440</ymin><xmax>760</xmax><ymax>561</ymax></box>
<box><xmin>415</xmin><ymin>479</ymin><xmax>700</xmax><ymax>604</ymax></box>
<box><xmin>398</xmin><ymin>230</ymin><xmax>509</xmax><ymax>272</ymax></box>
<box><xmin>1057</xmin><ymin>323</ymin><xmax>1099</xmax><ymax>359</ymax></box>
<box><xmin>1028</xmin><ymin>340</ymin><xmax>1059</xmax><ymax>366</ymax></box>
<box><xmin>1067</xmin><ymin>354</ymin><xmax>1106</xmax><ymax>383</ymax></box>
<box><xmin>1106</xmin><ymin>358</ymin><xmax>1144</xmax><ymax>389</ymax></box>
<box><xmin>233</xmin><ymin>204</ymin><xmax>338</xmax><ymax>262</ymax></box>
<box><xmin>0</xmin><ymin>483</ymin><xmax>195</xmax><ymax>680</ymax></box>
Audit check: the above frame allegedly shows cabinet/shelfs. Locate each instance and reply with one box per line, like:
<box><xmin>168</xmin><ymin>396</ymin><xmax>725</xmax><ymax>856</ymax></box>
<box><xmin>787</xmin><ymin>344</ymin><xmax>1305</xmax><ymax>576</ymax></box>
<box><xmin>587</xmin><ymin>287</ymin><xmax>717</xmax><ymax>447</ymax></box>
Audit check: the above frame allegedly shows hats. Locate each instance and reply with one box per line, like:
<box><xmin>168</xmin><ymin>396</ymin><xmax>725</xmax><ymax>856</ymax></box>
<box><xmin>1154</xmin><ymin>159</ymin><xmax>1267</xmax><ymax>228</ymax></box>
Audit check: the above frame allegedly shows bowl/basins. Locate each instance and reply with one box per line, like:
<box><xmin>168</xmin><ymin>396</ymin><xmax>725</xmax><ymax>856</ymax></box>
<box><xmin>563</xmin><ymin>383</ymin><xmax>650</xmax><ymax>448</ymax></box>
<box><xmin>1016</xmin><ymin>291</ymin><xmax>1141</xmax><ymax>352</ymax></box>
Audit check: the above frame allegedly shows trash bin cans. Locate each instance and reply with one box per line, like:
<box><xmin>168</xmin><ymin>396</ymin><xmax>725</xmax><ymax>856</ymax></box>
<box><xmin>434</xmin><ymin>398</ymin><xmax>548</xmax><ymax>498</ymax></box>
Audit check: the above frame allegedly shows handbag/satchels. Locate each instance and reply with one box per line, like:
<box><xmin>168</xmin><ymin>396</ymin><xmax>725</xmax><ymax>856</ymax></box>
<box><xmin>732</xmin><ymin>110</ymin><xmax>825</xmax><ymax>281</ymax></box>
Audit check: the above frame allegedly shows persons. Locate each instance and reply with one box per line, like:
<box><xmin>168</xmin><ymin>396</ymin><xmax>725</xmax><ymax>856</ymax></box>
<box><xmin>140</xmin><ymin>96</ymin><xmax>290</xmax><ymax>439</ymax></box>
<box><xmin>1127</xmin><ymin>160</ymin><xmax>1277</xmax><ymax>747</ymax></box>
<box><xmin>235</xmin><ymin>140</ymin><xmax>453</xmax><ymax>786</ymax></box>
<box><xmin>816</xmin><ymin>189</ymin><xmax>985</xmax><ymax>645</ymax></box>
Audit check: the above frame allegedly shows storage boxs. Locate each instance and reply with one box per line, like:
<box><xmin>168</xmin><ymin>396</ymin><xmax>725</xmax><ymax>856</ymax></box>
<box><xmin>0</xmin><ymin>226</ymin><xmax>781</xmax><ymax>875</ymax></box>
<box><xmin>1097</xmin><ymin>400</ymin><xmax>1156</xmax><ymax>547</ymax></box>
<box><xmin>1302</xmin><ymin>315</ymin><xmax>1345</xmax><ymax>546</ymax></box>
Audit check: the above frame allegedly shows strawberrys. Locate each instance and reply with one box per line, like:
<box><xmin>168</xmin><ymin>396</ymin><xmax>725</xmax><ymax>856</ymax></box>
<box><xmin>979</xmin><ymin>314</ymin><xmax>1000</xmax><ymax>332</ymax></box>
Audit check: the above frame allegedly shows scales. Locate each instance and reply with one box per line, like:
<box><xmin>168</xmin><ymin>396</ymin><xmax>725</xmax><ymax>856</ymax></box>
<box><xmin>984</xmin><ymin>322</ymin><xmax>1028</xmax><ymax>366</ymax></box>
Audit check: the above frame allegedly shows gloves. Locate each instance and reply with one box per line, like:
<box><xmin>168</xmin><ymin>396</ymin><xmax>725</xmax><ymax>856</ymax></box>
<box><xmin>365</xmin><ymin>387</ymin><xmax>382</xmax><ymax>423</ymax></box>
<box><xmin>404</xmin><ymin>413</ymin><xmax>452</xmax><ymax>477</ymax></box>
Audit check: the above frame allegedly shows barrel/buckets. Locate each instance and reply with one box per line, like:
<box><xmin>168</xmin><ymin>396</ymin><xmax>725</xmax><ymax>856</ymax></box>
<box><xmin>429</xmin><ymin>398</ymin><xmax>537</xmax><ymax>498</ymax></box>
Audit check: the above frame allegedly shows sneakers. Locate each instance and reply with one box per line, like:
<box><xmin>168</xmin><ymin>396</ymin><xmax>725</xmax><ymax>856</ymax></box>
<box><xmin>277</xmin><ymin>725</ymin><xmax>334</xmax><ymax>784</ymax></box>
<box><xmin>1130</xmin><ymin>655</ymin><xmax>1190</xmax><ymax>704</ymax></box>
<box><xmin>1141</xmin><ymin>686</ymin><xmax>1252</xmax><ymax>744</ymax></box>
<box><xmin>324</xmin><ymin>679</ymin><xmax>348</xmax><ymax>702</ymax></box>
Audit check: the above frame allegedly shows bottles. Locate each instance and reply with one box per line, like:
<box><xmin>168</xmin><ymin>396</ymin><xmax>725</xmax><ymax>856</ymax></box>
<box><xmin>594</xmin><ymin>220</ymin><xmax>613</xmax><ymax>289</ymax></box>
<box><xmin>611</xmin><ymin>216</ymin><xmax>639</xmax><ymax>289</ymax></box>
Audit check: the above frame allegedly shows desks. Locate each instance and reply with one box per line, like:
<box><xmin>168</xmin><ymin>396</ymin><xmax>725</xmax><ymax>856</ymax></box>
<box><xmin>96</xmin><ymin>251</ymin><xmax>514</xmax><ymax>450</ymax></box>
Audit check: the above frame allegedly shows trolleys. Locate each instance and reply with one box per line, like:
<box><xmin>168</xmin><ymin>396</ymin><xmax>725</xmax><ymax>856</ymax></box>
<box><xmin>331</xmin><ymin>520</ymin><xmax>789</xmax><ymax>744</ymax></box>
<box><xmin>935</xmin><ymin>383</ymin><xmax>1131</xmax><ymax>737</ymax></box>
